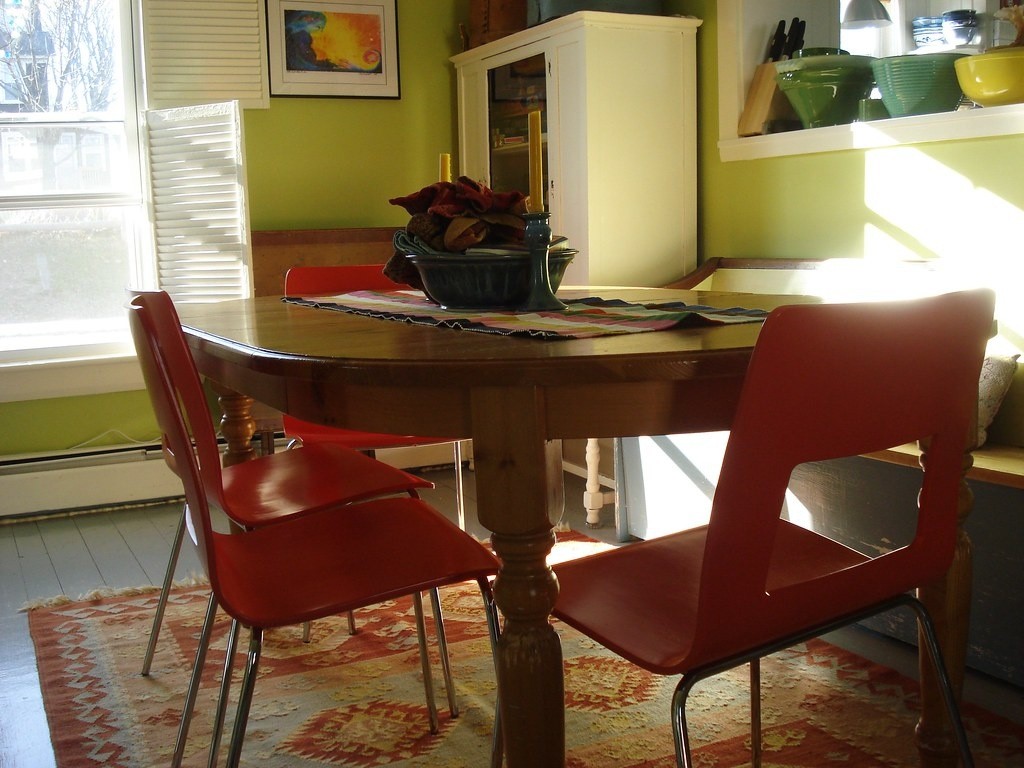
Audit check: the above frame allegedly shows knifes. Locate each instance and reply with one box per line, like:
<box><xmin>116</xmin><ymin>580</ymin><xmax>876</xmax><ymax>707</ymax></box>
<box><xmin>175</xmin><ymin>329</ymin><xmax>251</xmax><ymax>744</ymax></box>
<box><xmin>766</xmin><ymin>17</ymin><xmax>806</xmax><ymax>62</ymax></box>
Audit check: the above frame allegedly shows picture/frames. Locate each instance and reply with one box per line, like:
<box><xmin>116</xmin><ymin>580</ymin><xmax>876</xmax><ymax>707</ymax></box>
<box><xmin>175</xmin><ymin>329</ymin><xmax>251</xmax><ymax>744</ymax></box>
<box><xmin>265</xmin><ymin>0</ymin><xmax>402</xmax><ymax>101</ymax></box>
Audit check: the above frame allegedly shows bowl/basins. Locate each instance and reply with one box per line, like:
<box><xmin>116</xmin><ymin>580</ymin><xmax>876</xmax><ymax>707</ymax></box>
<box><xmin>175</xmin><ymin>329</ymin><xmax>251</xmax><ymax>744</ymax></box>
<box><xmin>773</xmin><ymin>55</ymin><xmax>878</xmax><ymax>128</ymax></box>
<box><xmin>405</xmin><ymin>248</ymin><xmax>579</xmax><ymax>312</ymax></box>
<box><xmin>792</xmin><ymin>48</ymin><xmax>850</xmax><ymax>58</ymax></box>
<box><xmin>871</xmin><ymin>53</ymin><xmax>973</xmax><ymax>118</ymax></box>
<box><xmin>912</xmin><ymin>9</ymin><xmax>977</xmax><ymax>48</ymax></box>
<box><xmin>954</xmin><ymin>47</ymin><xmax>1024</xmax><ymax>108</ymax></box>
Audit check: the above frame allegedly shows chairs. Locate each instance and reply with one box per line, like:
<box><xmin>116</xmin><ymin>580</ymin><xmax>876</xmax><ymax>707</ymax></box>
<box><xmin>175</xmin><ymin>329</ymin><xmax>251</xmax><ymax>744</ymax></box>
<box><xmin>126</xmin><ymin>294</ymin><xmax>505</xmax><ymax>768</ymax></box>
<box><xmin>139</xmin><ymin>288</ymin><xmax>444</xmax><ymax>736</ymax></box>
<box><xmin>490</xmin><ymin>288</ymin><xmax>996</xmax><ymax>768</ymax></box>
<box><xmin>284</xmin><ymin>262</ymin><xmax>414</xmax><ymax>637</ymax></box>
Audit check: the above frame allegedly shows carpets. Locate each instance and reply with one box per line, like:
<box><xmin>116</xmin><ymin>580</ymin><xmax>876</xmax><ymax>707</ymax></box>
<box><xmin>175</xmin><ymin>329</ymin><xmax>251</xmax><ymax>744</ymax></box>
<box><xmin>15</xmin><ymin>528</ymin><xmax>1024</xmax><ymax>768</ymax></box>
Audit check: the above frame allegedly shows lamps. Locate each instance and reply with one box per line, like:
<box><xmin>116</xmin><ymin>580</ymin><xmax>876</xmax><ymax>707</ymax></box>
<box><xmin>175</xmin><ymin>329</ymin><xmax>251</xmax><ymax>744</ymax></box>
<box><xmin>842</xmin><ymin>0</ymin><xmax>894</xmax><ymax>30</ymax></box>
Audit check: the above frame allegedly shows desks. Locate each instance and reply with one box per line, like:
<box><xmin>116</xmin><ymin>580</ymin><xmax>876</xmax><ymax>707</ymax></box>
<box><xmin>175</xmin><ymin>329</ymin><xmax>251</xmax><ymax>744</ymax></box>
<box><xmin>174</xmin><ymin>284</ymin><xmax>826</xmax><ymax>768</ymax></box>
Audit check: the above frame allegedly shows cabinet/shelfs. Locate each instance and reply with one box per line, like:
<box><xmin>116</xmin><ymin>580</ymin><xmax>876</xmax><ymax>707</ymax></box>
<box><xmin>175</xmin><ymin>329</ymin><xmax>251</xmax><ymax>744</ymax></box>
<box><xmin>447</xmin><ymin>10</ymin><xmax>708</xmax><ymax>492</ymax></box>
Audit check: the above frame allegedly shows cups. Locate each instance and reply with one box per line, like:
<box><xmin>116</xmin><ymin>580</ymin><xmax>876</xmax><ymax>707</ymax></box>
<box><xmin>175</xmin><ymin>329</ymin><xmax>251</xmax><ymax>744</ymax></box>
<box><xmin>859</xmin><ymin>99</ymin><xmax>891</xmax><ymax>121</ymax></box>
